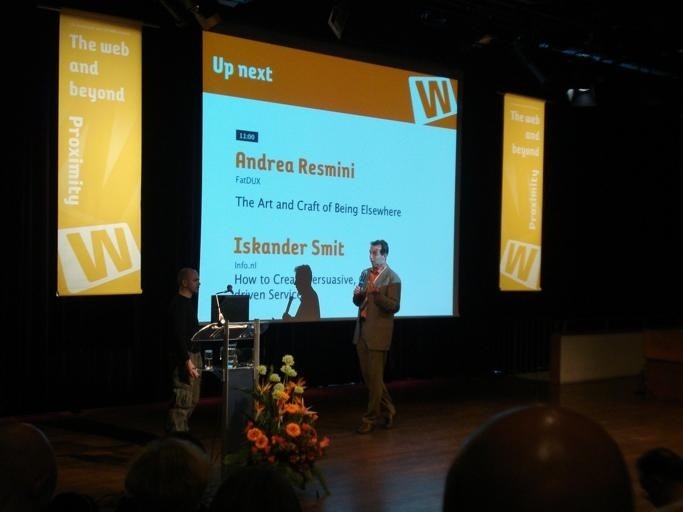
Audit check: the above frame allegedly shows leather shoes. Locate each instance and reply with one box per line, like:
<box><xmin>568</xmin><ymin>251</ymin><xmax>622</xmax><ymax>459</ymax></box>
<box><xmin>382</xmin><ymin>413</ymin><xmax>396</xmax><ymax>430</ymax></box>
<box><xmin>356</xmin><ymin>421</ymin><xmax>376</xmax><ymax>433</ymax></box>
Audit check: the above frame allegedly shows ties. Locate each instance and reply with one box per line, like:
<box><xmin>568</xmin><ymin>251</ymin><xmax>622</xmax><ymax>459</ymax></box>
<box><xmin>361</xmin><ymin>271</ymin><xmax>378</xmax><ymax>319</ymax></box>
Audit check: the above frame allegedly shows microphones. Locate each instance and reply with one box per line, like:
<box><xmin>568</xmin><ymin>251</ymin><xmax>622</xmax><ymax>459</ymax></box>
<box><xmin>227</xmin><ymin>284</ymin><xmax>234</xmax><ymax>294</ymax></box>
<box><xmin>359</xmin><ymin>272</ymin><xmax>366</xmax><ymax>288</ymax></box>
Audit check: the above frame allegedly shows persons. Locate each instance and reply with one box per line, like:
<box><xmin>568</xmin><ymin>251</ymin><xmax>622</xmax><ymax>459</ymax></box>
<box><xmin>637</xmin><ymin>447</ymin><xmax>683</xmax><ymax>512</ymax></box>
<box><xmin>158</xmin><ymin>268</ymin><xmax>203</xmax><ymax>432</ymax></box>
<box><xmin>351</xmin><ymin>239</ymin><xmax>401</xmax><ymax>435</ymax></box>
<box><xmin>443</xmin><ymin>404</ymin><xmax>635</xmax><ymax>510</ymax></box>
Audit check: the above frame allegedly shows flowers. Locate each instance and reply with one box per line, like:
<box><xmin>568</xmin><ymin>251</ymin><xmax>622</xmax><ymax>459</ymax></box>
<box><xmin>221</xmin><ymin>348</ymin><xmax>331</xmax><ymax>495</ymax></box>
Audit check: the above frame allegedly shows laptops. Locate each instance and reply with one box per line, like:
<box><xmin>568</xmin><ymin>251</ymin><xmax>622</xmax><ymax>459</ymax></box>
<box><xmin>210</xmin><ymin>295</ymin><xmax>250</xmax><ymax>322</ymax></box>
